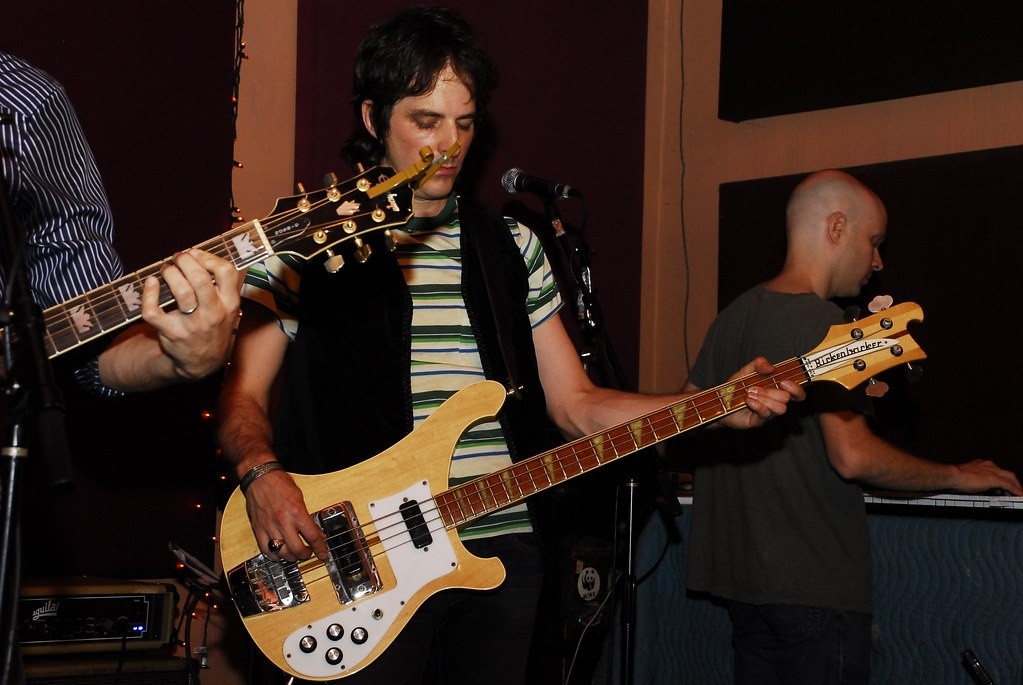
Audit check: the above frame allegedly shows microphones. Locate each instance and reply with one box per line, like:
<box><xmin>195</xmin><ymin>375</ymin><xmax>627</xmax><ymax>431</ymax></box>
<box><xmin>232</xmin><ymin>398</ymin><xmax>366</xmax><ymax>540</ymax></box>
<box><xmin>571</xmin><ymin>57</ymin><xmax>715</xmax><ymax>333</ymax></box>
<box><xmin>501</xmin><ymin>168</ymin><xmax>583</xmax><ymax>198</ymax></box>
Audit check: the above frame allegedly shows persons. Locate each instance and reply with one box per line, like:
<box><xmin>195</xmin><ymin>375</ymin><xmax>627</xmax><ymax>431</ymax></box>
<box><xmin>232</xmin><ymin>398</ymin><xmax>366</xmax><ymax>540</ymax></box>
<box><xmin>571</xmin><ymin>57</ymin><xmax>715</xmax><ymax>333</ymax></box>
<box><xmin>0</xmin><ymin>42</ymin><xmax>242</xmax><ymax>409</ymax></box>
<box><xmin>671</xmin><ymin>168</ymin><xmax>1023</xmax><ymax>685</ymax></box>
<box><xmin>216</xmin><ymin>7</ymin><xmax>808</xmax><ymax>685</ymax></box>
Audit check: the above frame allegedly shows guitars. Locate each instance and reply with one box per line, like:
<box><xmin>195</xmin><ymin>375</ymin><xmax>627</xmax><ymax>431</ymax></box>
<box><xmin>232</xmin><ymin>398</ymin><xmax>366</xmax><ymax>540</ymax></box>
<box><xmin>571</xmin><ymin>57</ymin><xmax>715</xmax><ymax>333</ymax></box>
<box><xmin>212</xmin><ymin>294</ymin><xmax>932</xmax><ymax>682</ymax></box>
<box><xmin>0</xmin><ymin>141</ymin><xmax>463</xmax><ymax>377</ymax></box>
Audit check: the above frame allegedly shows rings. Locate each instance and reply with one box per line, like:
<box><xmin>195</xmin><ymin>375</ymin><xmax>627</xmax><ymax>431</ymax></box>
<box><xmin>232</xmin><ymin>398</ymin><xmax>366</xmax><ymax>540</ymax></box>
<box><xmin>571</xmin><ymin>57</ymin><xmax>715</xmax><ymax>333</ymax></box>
<box><xmin>178</xmin><ymin>303</ymin><xmax>200</xmax><ymax>314</ymax></box>
<box><xmin>267</xmin><ymin>537</ymin><xmax>286</xmax><ymax>554</ymax></box>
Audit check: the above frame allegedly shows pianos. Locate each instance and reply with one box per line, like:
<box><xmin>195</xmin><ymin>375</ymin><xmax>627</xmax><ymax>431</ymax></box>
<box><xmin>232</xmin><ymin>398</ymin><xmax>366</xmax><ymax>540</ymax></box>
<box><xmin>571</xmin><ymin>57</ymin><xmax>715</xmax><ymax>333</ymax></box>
<box><xmin>618</xmin><ymin>485</ymin><xmax>1023</xmax><ymax>685</ymax></box>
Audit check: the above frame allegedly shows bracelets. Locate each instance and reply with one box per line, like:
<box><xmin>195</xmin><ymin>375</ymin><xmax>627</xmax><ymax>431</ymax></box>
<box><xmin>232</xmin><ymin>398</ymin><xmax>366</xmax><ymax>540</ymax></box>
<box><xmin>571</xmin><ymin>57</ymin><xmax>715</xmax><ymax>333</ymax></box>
<box><xmin>238</xmin><ymin>459</ymin><xmax>288</xmax><ymax>498</ymax></box>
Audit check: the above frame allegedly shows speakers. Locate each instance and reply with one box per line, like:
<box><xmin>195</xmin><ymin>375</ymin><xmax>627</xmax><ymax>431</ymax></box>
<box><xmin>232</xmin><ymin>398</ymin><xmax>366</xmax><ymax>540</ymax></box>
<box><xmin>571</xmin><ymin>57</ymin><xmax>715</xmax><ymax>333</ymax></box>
<box><xmin>18</xmin><ymin>656</ymin><xmax>200</xmax><ymax>685</ymax></box>
